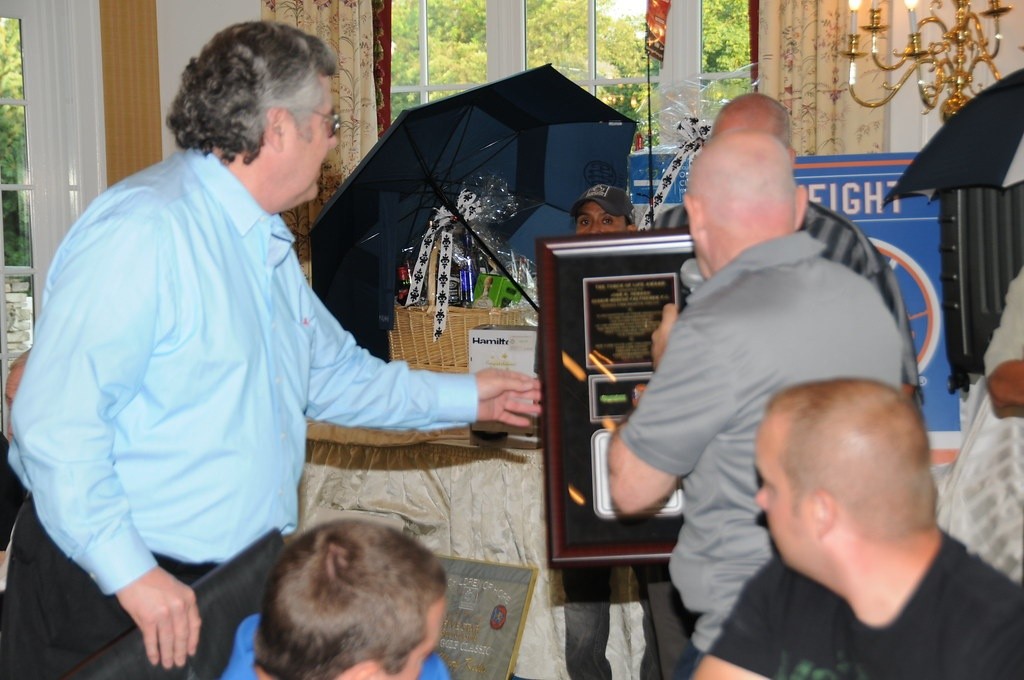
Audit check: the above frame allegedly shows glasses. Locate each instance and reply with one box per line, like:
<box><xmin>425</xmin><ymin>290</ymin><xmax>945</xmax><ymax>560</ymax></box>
<box><xmin>298</xmin><ymin>104</ymin><xmax>341</xmax><ymax>137</ymax></box>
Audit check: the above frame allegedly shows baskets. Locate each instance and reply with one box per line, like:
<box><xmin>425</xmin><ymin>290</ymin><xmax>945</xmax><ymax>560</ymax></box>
<box><xmin>386</xmin><ymin>237</ymin><xmax>528</xmax><ymax>372</ymax></box>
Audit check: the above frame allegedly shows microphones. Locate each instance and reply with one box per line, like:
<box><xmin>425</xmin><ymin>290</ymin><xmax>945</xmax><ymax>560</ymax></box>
<box><xmin>678</xmin><ymin>258</ymin><xmax>704</xmax><ymax>315</ymax></box>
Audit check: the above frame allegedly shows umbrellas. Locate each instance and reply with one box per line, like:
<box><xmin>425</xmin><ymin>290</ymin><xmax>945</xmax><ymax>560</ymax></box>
<box><xmin>307</xmin><ymin>61</ymin><xmax>639</xmax><ymax>363</ymax></box>
<box><xmin>883</xmin><ymin>66</ymin><xmax>1023</xmax><ymax>204</ymax></box>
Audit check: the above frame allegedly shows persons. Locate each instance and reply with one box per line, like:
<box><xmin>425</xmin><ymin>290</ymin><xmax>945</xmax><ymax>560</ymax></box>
<box><xmin>964</xmin><ymin>138</ymin><xmax>1024</xmax><ymax>592</ymax></box>
<box><xmin>564</xmin><ymin>183</ymin><xmax>662</xmax><ymax>680</ymax></box>
<box><xmin>651</xmin><ymin>92</ymin><xmax>916</xmax><ymax>371</ymax></box>
<box><xmin>0</xmin><ymin>18</ymin><xmax>545</xmax><ymax>680</ymax></box>
<box><xmin>690</xmin><ymin>377</ymin><xmax>1024</xmax><ymax>679</ymax></box>
<box><xmin>221</xmin><ymin>519</ymin><xmax>452</xmax><ymax>679</ymax></box>
<box><xmin>607</xmin><ymin>127</ymin><xmax>903</xmax><ymax>680</ymax></box>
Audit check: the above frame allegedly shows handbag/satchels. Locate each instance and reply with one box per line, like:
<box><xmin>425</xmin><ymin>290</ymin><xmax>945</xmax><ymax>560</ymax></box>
<box><xmin>933</xmin><ymin>388</ymin><xmax>1024</xmax><ymax>586</ymax></box>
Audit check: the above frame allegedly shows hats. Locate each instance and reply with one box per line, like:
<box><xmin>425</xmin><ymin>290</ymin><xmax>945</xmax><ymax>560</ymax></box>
<box><xmin>570</xmin><ymin>184</ymin><xmax>635</xmax><ymax>224</ymax></box>
